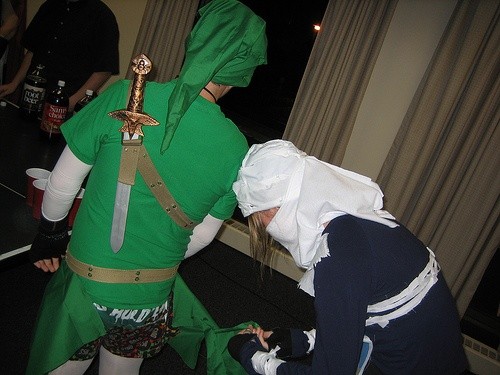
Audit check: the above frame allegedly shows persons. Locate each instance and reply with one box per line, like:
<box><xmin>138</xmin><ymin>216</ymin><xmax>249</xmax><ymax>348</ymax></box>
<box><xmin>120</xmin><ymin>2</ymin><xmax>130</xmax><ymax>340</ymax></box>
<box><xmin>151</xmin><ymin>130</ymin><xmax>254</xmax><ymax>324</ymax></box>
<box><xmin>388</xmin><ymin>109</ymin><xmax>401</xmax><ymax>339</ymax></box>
<box><xmin>0</xmin><ymin>0</ymin><xmax>121</xmax><ymax>113</ymax></box>
<box><xmin>0</xmin><ymin>0</ymin><xmax>20</xmax><ymax>84</ymax></box>
<box><xmin>21</xmin><ymin>0</ymin><xmax>269</xmax><ymax>375</ymax></box>
<box><xmin>228</xmin><ymin>138</ymin><xmax>473</xmax><ymax>375</ymax></box>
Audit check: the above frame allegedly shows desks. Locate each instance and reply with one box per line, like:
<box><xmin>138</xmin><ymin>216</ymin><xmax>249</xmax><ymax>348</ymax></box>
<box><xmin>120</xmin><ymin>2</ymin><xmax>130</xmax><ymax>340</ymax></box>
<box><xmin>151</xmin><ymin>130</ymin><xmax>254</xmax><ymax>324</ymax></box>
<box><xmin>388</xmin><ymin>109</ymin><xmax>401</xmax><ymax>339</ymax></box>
<box><xmin>0</xmin><ymin>182</ymin><xmax>73</xmax><ymax>261</ymax></box>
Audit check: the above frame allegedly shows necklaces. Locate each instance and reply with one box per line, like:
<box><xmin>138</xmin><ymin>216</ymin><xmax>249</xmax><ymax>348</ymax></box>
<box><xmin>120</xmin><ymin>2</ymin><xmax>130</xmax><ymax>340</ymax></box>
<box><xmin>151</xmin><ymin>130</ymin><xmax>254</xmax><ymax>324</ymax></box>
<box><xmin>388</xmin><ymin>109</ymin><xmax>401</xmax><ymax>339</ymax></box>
<box><xmin>174</xmin><ymin>74</ymin><xmax>217</xmax><ymax>105</ymax></box>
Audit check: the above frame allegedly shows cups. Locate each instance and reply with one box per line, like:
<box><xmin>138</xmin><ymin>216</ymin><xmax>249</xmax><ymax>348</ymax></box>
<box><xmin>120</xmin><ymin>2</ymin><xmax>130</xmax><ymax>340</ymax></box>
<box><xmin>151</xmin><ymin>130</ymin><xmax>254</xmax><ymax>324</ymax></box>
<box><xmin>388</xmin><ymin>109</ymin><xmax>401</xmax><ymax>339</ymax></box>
<box><xmin>31</xmin><ymin>179</ymin><xmax>49</xmax><ymax>220</ymax></box>
<box><xmin>25</xmin><ymin>167</ymin><xmax>51</xmax><ymax>207</ymax></box>
<box><xmin>66</xmin><ymin>187</ymin><xmax>86</xmax><ymax>228</ymax></box>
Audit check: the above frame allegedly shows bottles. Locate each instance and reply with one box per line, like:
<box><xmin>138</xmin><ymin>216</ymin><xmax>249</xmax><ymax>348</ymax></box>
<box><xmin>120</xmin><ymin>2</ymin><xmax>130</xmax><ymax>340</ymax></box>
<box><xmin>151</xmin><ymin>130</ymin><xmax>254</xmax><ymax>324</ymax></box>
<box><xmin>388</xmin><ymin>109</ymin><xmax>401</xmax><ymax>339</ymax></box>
<box><xmin>71</xmin><ymin>89</ymin><xmax>97</xmax><ymax>117</ymax></box>
<box><xmin>19</xmin><ymin>64</ymin><xmax>47</xmax><ymax>115</ymax></box>
<box><xmin>39</xmin><ymin>80</ymin><xmax>70</xmax><ymax>147</ymax></box>
